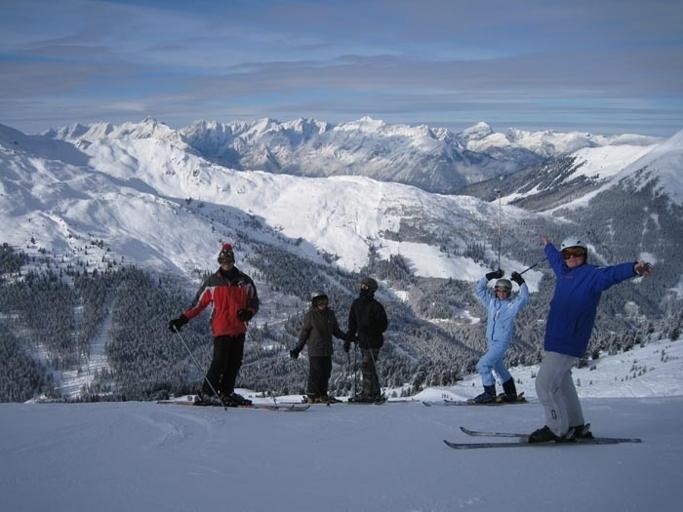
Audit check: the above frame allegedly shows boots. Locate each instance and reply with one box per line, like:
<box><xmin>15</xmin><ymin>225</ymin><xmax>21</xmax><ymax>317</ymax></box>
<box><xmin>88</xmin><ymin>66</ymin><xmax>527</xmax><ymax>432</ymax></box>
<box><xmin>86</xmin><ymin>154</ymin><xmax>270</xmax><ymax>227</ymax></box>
<box><xmin>497</xmin><ymin>378</ymin><xmax>517</xmax><ymax>400</ymax></box>
<box><xmin>475</xmin><ymin>384</ymin><xmax>496</xmax><ymax>401</ymax></box>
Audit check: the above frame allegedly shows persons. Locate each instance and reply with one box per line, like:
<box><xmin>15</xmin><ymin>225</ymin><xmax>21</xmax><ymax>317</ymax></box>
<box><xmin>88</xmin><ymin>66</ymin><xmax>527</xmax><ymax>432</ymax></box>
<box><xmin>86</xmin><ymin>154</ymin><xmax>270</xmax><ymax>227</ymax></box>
<box><xmin>289</xmin><ymin>288</ymin><xmax>356</xmax><ymax>402</ymax></box>
<box><xmin>169</xmin><ymin>243</ymin><xmax>260</xmax><ymax>407</ymax></box>
<box><xmin>524</xmin><ymin>231</ymin><xmax>653</xmax><ymax>443</ymax></box>
<box><xmin>474</xmin><ymin>269</ymin><xmax>530</xmax><ymax>403</ymax></box>
<box><xmin>342</xmin><ymin>279</ymin><xmax>388</xmax><ymax>401</ymax></box>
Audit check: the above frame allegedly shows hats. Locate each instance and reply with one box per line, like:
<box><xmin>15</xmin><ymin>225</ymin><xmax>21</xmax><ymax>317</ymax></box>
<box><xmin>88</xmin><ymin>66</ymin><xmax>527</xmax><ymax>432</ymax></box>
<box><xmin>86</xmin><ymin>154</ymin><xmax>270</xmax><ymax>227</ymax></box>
<box><xmin>218</xmin><ymin>244</ymin><xmax>235</xmax><ymax>263</ymax></box>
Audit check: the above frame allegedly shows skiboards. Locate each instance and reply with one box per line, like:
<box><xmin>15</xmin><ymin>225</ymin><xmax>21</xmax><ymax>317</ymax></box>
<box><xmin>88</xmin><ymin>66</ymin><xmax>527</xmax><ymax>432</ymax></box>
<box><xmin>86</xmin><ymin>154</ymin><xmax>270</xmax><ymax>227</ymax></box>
<box><xmin>281</xmin><ymin>397</ymin><xmax>389</xmax><ymax>406</ymax></box>
<box><xmin>155</xmin><ymin>400</ymin><xmax>311</xmax><ymax>412</ymax></box>
<box><xmin>443</xmin><ymin>426</ymin><xmax>641</xmax><ymax>449</ymax></box>
<box><xmin>422</xmin><ymin>398</ymin><xmax>527</xmax><ymax>407</ymax></box>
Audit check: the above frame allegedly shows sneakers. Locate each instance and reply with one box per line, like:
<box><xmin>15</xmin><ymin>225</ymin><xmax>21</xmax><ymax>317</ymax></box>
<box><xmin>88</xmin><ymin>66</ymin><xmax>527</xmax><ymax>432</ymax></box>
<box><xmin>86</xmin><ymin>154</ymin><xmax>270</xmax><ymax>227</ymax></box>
<box><xmin>353</xmin><ymin>394</ymin><xmax>380</xmax><ymax>400</ymax></box>
<box><xmin>528</xmin><ymin>426</ymin><xmax>570</xmax><ymax>442</ymax></box>
<box><xmin>221</xmin><ymin>392</ymin><xmax>252</xmax><ymax>405</ymax></box>
<box><xmin>307</xmin><ymin>393</ymin><xmax>335</xmax><ymax>401</ymax></box>
<box><xmin>570</xmin><ymin>424</ymin><xmax>588</xmax><ymax>438</ymax></box>
<box><xmin>200</xmin><ymin>391</ymin><xmax>230</xmax><ymax>403</ymax></box>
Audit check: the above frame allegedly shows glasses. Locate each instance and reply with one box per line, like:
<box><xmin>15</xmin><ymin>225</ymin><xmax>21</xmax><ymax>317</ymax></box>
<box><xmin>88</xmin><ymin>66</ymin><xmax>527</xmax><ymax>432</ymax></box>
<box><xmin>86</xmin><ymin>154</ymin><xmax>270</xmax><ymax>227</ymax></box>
<box><xmin>362</xmin><ymin>285</ymin><xmax>369</xmax><ymax>291</ymax></box>
<box><xmin>315</xmin><ymin>298</ymin><xmax>327</xmax><ymax>305</ymax></box>
<box><xmin>563</xmin><ymin>248</ymin><xmax>584</xmax><ymax>260</ymax></box>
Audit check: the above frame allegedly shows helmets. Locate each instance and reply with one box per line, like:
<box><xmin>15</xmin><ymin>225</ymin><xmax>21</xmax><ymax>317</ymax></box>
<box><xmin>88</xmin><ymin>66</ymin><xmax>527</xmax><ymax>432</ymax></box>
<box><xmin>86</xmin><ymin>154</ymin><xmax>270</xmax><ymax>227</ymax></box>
<box><xmin>561</xmin><ymin>237</ymin><xmax>587</xmax><ymax>251</ymax></box>
<box><xmin>495</xmin><ymin>279</ymin><xmax>511</xmax><ymax>298</ymax></box>
<box><xmin>360</xmin><ymin>278</ymin><xmax>378</xmax><ymax>295</ymax></box>
<box><xmin>310</xmin><ymin>291</ymin><xmax>329</xmax><ymax>307</ymax></box>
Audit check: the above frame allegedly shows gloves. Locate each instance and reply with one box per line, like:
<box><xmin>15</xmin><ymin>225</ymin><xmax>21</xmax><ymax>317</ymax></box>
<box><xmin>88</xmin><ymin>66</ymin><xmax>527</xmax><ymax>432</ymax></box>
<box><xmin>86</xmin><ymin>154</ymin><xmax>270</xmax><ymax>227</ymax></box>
<box><xmin>342</xmin><ymin>334</ymin><xmax>358</xmax><ymax>342</ymax></box>
<box><xmin>486</xmin><ymin>270</ymin><xmax>504</xmax><ymax>280</ymax></box>
<box><xmin>169</xmin><ymin>314</ymin><xmax>188</xmax><ymax>333</ymax></box>
<box><xmin>237</xmin><ymin>309</ymin><xmax>251</xmax><ymax>321</ymax></box>
<box><xmin>511</xmin><ymin>272</ymin><xmax>525</xmax><ymax>286</ymax></box>
<box><xmin>290</xmin><ymin>349</ymin><xmax>299</xmax><ymax>359</ymax></box>
<box><xmin>343</xmin><ymin>342</ymin><xmax>351</xmax><ymax>352</ymax></box>
